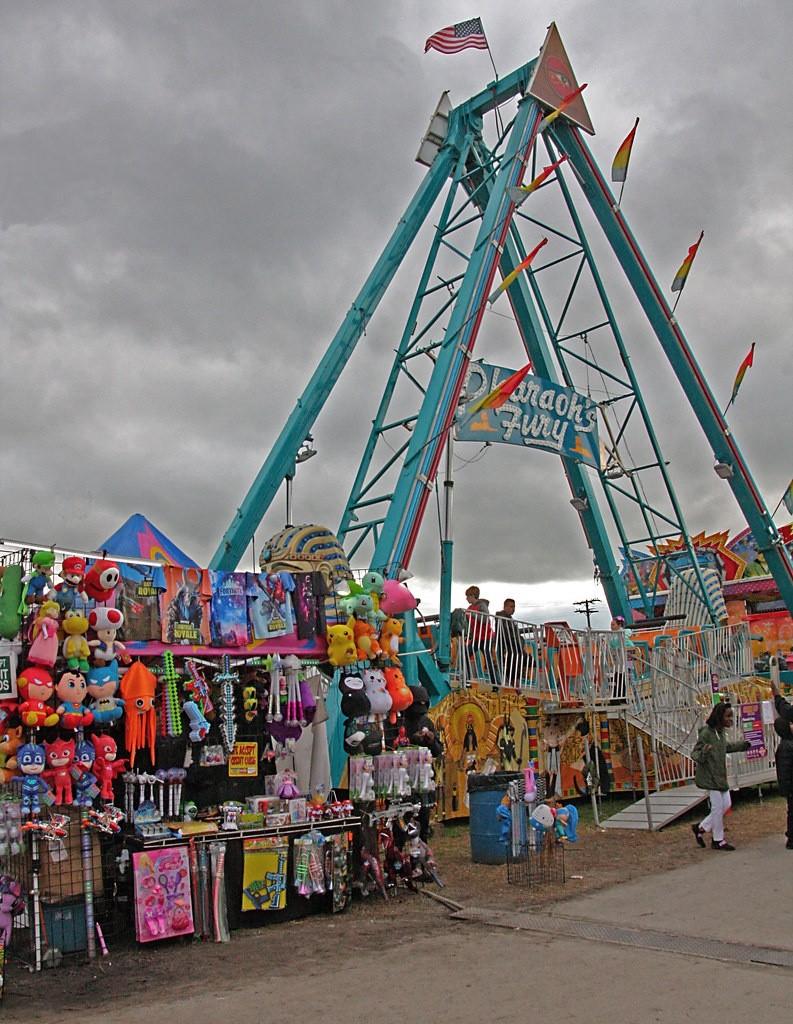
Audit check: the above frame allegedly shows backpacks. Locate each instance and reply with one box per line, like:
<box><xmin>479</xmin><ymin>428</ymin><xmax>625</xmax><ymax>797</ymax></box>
<box><xmin>451</xmin><ymin>608</ymin><xmax>468</xmax><ymax>638</ymax></box>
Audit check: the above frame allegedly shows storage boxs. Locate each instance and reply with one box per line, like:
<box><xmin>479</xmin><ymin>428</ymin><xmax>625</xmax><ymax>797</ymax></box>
<box><xmin>284</xmin><ymin>798</ymin><xmax>307</xmax><ymax>824</ymax></box>
<box><xmin>264</xmin><ymin>812</ymin><xmax>290</xmax><ymax>828</ymax></box>
<box><xmin>29</xmin><ymin>902</ymin><xmax>87</xmax><ymax>954</ymax></box>
<box><xmin>246</xmin><ymin>794</ymin><xmax>279</xmax><ymax>814</ymax></box>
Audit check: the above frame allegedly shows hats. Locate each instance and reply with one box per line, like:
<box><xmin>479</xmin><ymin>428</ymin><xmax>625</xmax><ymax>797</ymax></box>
<box><xmin>343</xmin><ymin>717</ymin><xmax>365</xmax><ymax>754</ymax></box>
<box><xmin>361</xmin><ymin>722</ymin><xmax>383</xmax><ymax>756</ymax></box>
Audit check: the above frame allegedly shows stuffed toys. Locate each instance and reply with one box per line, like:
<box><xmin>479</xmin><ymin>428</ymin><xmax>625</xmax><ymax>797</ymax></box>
<box><xmin>338</xmin><ymin>667</ymin><xmax>413</xmax><ymax>724</ymax></box>
<box><xmin>27</xmin><ymin>600</ymin><xmax>125</xmax><ymax>673</ymax></box>
<box><xmin>0</xmin><ymin>876</ymin><xmax>25</xmax><ymax>946</ymax></box>
<box><xmin>17</xmin><ymin>659</ymin><xmax>125</xmax><ymax>729</ymax></box>
<box><xmin>326</xmin><ymin>572</ymin><xmax>417</xmax><ymax>666</ymax></box>
<box><xmin>11</xmin><ymin>733</ymin><xmax>125</xmax><ymax>813</ymax></box>
<box><xmin>0</xmin><ymin>700</ymin><xmax>28</xmax><ymax>785</ymax></box>
<box><xmin>21</xmin><ymin>551</ymin><xmax>121</xmax><ymax>612</ymax></box>
<box><xmin>120</xmin><ymin>662</ymin><xmax>157</xmax><ymax>768</ymax></box>
<box><xmin>529</xmin><ymin>804</ymin><xmax>579</xmax><ymax>844</ymax></box>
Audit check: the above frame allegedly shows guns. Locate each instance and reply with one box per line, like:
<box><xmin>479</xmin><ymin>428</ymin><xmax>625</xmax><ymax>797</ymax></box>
<box><xmin>182</xmin><ymin>701</ymin><xmax>212</xmax><ymax>741</ymax></box>
<box><xmin>183</xmin><ymin>660</ymin><xmax>214</xmax><ymax>715</ymax></box>
<box><xmin>364</xmin><ymin>798</ymin><xmax>439</xmax><ymax>832</ymax></box>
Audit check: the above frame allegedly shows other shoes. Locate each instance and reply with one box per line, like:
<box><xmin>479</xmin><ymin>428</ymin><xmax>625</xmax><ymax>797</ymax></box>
<box><xmin>608</xmin><ymin>702</ymin><xmax>621</xmax><ymax>706</ymax></box>
<box><xmin>691</xmin><ymin>822</ymin><xmax>706</xmax><ymax>848</ymax></box>
<box><xmin>491</xmin><ymin>682</ymin><xmax>501</xmax><ymax>692</ymax></box>
<box><xmin>458</xmin><ymin>683</ymin><xmax>472</xmax><ymax>688</ymax></box>
<box><xmin>711</xmin><ymin>836</ymin><xmax>735</xmax><ymax>851</ymax></box>
<box><xmin>786</xmin><ymin>838</ymin><xmax>793</xmax><ymax>849</ymax></box>
<box><xmin>519</xmin><ymin>694</ymin><xmax>525</xmax><ymax>697</ymax></box>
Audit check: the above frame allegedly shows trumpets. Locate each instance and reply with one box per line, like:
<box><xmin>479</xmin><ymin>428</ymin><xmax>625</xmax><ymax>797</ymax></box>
<box><xmin>296</xmin><ymin>844</ymin><xmax>334</xmax><ymax>894</ymax></box>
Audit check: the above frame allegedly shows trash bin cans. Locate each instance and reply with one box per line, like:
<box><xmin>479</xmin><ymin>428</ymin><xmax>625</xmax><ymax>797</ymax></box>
<box><xmin>468</xmin><ymin>770</ymin><xmax>525</xmax><ymax>865</ymax></box>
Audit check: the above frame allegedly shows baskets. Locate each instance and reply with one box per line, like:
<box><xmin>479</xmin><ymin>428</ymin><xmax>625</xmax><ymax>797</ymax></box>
<box><xmin>507</xmin><ymin>843</ymin><xmax>565</xmax><ymax>892</ymax></box>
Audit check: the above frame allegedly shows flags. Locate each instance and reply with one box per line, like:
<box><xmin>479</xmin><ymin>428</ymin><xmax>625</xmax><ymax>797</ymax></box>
<box><xmin>671</xmin><ymin>230</ymin><xmax>704</xmax><ymax>291</ymax></box>
<box><xmin>732</xmin><ymin>342</ymin><xmax>754</xmax><ymax>403</ymax></box>
<box><xmin>423</xmin><ymin>17</ymin><xmax>489</xmax><ymax>54</ymax></box>
<box><xmin>782</xmin><ymin>479</ymin><xmax>793</xmax><ymax>516</ymax></box>
<box><xmin>468</xmin><ymin>362</ymin><xmax>532</xmax><ymax>413</ymax></box>
<box><xmin>534</xmin><ymin>83</ymin><xmax>587</xmax><ymax>133</ymax></box>
<box><xmin>611</xmin><ymin>117</ymin><xmax>639</xmax><ymax>181</ymax></box>
<box><xmin>486</xmin><ymin>237</ymin><xmax>548</xmax><ymax>304</ymax></box>
<box><xmin>505</xmin><ymin>153</ymin><xmax>567</xmax><ymax>203</ymax></box>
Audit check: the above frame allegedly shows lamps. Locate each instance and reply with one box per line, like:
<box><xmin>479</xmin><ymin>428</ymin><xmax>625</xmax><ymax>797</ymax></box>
<box><xmin>714</xmin><ymin>463</ymin><xmax>735</xmax><ymax>479</ymax></box>
<box><xmin>569</xmin><ymin>498</ymin><xmax>589</xmax><ymax>512</ymax></box>
<box><xmin>296</xmin><ymin>444</ymin><xmax>317</xmax><ymax>463</ymax></box>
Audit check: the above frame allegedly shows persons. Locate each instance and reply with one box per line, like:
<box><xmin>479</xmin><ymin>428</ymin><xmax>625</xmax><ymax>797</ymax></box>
<box><xmin>495</xmin><ymin>599</ymin><xmax>537</xmax><ymax>697</ymax></box>
<box><xmin>690</xmin><ymin>703</ymin><xmax>752</xmax><ymax>851</ymax></box>
<box><xmin>461</xmin><ymin>586</ymin><xmax>500</xmax><ymax>693</ymax></box>
<box><xmin>402</xmin><ymin>685</ymin><xmax>441</xmax><ymax>844</ymax></box>
<box><xmin>607</xmin><ymin>616</ymin><xmax>634</xmax><ymax>705</ymax></box>
<box><xmin>769</xmin><ymin>679</ymin><xmax>793</xmax><ymax>850</ymax></box>
